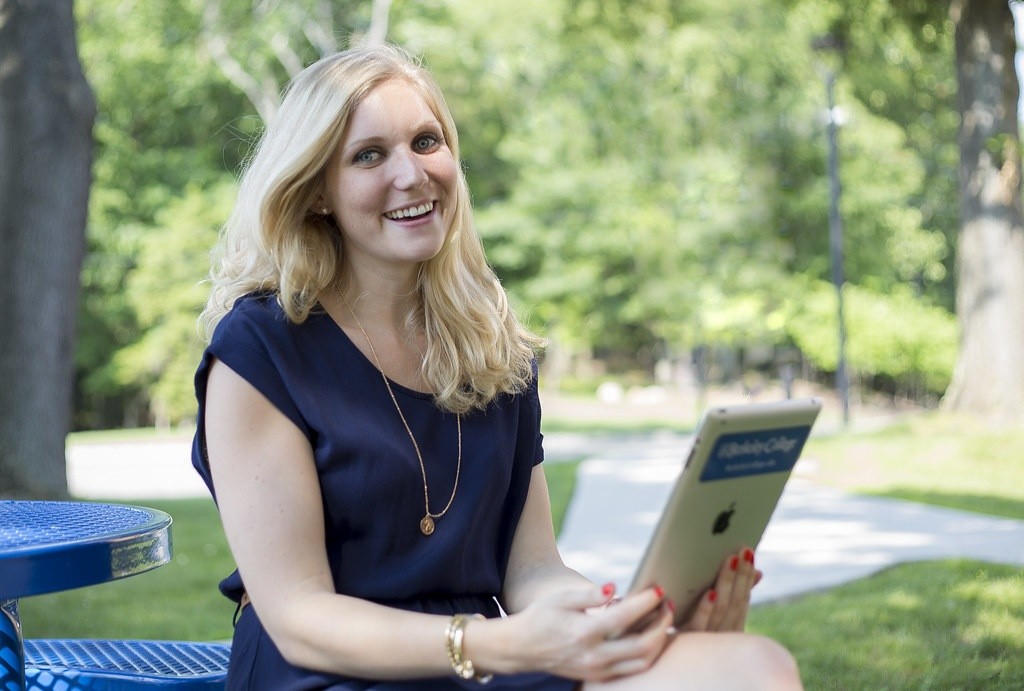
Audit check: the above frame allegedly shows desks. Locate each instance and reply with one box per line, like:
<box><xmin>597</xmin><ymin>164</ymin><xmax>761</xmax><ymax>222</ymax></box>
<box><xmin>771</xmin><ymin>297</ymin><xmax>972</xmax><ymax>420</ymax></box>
<box><xmin>0</xmin><ymin>499</ymin><xmax>172</xmax><ymax>691</ymax></box>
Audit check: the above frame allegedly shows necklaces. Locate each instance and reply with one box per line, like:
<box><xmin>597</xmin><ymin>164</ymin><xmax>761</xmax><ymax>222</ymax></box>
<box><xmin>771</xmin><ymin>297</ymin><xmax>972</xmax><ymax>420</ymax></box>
<box><xmin>333</xmin><ymin>279</ymin><xmax>461</xmax><ymax>535</ymax></box>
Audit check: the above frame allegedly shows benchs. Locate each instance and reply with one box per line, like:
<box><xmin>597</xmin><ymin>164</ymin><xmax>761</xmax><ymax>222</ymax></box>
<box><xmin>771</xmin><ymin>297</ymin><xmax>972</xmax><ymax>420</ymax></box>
<box><xmin>23</xmin><ymin>640</ymin><xmax>232</xmax><ymax>691</ymax></box>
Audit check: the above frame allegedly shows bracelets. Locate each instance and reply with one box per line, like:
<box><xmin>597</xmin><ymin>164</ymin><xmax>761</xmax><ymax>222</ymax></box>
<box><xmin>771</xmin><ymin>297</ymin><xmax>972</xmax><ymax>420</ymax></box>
<box><xmin>445</xmin><ymin>610</ymin><xmax>494</xmax><ymax>684</ymax></box>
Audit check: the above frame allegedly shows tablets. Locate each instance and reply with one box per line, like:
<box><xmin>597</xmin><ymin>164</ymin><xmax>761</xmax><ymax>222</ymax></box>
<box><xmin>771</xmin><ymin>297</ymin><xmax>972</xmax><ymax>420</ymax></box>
<box><xmin>626</xmin><ymin>395</ymin><xmax>825</xmax><ymax>624</ymax></box>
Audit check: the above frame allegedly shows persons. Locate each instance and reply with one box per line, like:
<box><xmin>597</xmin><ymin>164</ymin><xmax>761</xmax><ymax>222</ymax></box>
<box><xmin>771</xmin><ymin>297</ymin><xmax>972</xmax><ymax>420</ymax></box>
<box><xmin>190</xmin><ymin>42</ymin><xmax>804</xmax><ymax>691</ymax></box>
